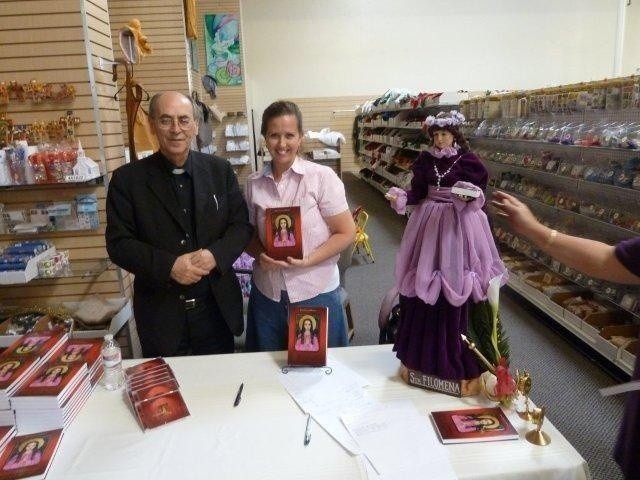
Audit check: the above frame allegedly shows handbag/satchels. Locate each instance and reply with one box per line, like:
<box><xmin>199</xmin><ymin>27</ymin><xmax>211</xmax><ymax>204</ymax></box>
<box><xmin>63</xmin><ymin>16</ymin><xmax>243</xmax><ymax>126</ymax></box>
<box><xmin>191</xmin><ymin>91</ymin><xmax>212</xmax><ymax>148</ymax></box>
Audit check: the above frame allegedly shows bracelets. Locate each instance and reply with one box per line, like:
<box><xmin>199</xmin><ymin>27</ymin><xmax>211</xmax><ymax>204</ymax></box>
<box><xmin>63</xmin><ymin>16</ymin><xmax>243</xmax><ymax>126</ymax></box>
<box><xmin>539</xmin><ymin>228</ymin><xmax>559</xmax><ymax>255</ymax></box>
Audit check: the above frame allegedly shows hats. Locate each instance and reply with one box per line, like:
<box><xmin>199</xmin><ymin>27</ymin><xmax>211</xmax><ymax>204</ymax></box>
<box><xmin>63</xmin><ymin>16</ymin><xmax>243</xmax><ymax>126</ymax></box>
<box><xmin>275</xmin><ymin>214</ymin><xmax>292</xmax><ymax>228</ymax></box>
<box><xmin>19</xmin><ymin>438</ymin><xmax>44</xmax><ymax>451</ymax></box>
<box><xmin>17</xmin><ymin>346</ymin><xmax>37</xmax><ymax>354</ymax></box>
<box><xmin>61</xmin><ymin>354</ymin><xmax>81</xmax><ymax>362</ymax></box>
<box><xmin>46</xmin><ymin>365</ymin><xmax>68</xmax><ymax>376</ymax></box>
<box><xmin>478</xmin><ymin>415</ymin><xmax>499</xmax><ymax>429</ymax></box>
<box><xmin>299</xmin><ymin>315</ymin><xmax>316</xmax><ymax>331</ymax></box>
<box><xmin>0</xmin><ymin>360</ymin><xmax>20</xmax><ymax>369</ymax></box>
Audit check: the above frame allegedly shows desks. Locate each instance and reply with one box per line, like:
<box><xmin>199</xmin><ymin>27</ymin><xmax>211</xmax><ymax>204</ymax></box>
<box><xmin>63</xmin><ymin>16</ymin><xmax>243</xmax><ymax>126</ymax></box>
<box><xmin>38</xmin><ymin>343</ymin><xmax>588</xmax><ymax>478</ymax></box>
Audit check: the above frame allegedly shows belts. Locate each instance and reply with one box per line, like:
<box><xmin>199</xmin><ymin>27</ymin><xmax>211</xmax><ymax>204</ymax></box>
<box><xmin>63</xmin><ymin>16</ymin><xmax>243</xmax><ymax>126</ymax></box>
<box><xmin>186</xmin><ymin>296</ymin><xmax>206</xmax><ymax>310</ymax></box>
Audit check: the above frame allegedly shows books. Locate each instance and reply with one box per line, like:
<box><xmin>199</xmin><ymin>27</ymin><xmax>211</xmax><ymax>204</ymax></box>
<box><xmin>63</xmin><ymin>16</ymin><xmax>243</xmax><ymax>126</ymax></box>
<box><xmin>266</xmin><ymin>208</ymin><xmax>304</xmax><ymax>263</ymax></box>
<box><xmin>121</xmin><ymin>354</ymin><xmax>193</xmax><ymax>434</ymax></box>
<box><xmin>429</xmin><ymin>406</ymin><xmax>520</xmax><ymax>444</ymax></box>
<box><xmin>0</xmin><ymin>332</ymin><xmax>109</xmax><ymax>480</ymax></box>
<box><xmin>286</xmin><ymin>305</ymin><xmax>329</xmax><ymax>369</ymax></box>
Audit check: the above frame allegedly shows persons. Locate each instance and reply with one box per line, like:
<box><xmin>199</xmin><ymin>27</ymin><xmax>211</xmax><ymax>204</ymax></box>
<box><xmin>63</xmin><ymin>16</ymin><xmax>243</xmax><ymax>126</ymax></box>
<box><xmin>381</xmin><ymin>106</ymin><xmax>512</xmax><ymax>396</ymax></box>
<box><xmin>105</xmin><ymin>89</ymin><xmax>254</xmax><ymax>358</ymax></box>
<box><xmin>452</xmin><ymin>412</ymin><xmax>509</xmax><ymax>432</ymax></box>
<box><xmin>488</xmin><ymin>186</ymin><xmax>640</xmax><ymax>480</ymax></box>
<box><xmin>272</xmin><ymin>214</ymin><xmax>298</xmax><ymax>247</ymax></box>
<box><xmin>296</xmin><ymin>315</ymin><xmax>320</xmax><ymax>351</ymax></box>
<box><xmin>238</xmin><ymin>99</ymin><xmax>358</xmax><ymax>353</ymax></box>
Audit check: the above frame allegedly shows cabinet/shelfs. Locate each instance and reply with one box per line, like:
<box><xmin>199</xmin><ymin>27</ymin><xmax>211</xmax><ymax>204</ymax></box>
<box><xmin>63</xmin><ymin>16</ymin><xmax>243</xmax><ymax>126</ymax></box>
<box><xmin>0</xmin><ymin>176</ymin><xmax>132</xmax><ymax>341</ymax></box>
<box><xmin>353</xmin><ymin>78</ymin><xmax>639</xmax><ymax>388</ymax></box>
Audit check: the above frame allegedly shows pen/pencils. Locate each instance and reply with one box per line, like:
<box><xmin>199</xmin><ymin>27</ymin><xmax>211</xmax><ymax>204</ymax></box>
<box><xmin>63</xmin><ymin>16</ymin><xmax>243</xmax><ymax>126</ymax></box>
<box><xmin>304</xmin><ymin>414</ymin><xmax>312</xmax><ymax>445</ymax></box>
<box><xmin>234</xmin><ymin>383</ymin><xmax>244</xmax><ymax>406</ymax></box>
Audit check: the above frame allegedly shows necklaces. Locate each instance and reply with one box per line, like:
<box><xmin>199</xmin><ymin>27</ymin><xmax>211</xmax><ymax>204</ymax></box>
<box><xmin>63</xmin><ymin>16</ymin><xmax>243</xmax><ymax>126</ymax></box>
<box><xmin>433</xmin><ymin>155</ymin><xmax>464</xmax><ymax>190</ymax></box>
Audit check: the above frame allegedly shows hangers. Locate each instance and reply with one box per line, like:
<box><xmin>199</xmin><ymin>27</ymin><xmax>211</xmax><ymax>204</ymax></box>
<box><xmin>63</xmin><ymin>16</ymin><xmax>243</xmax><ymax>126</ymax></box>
<box><xmin>114</xmin><ymin>60</ymin><xmax>149</xmax><ymax>103</ymax></box>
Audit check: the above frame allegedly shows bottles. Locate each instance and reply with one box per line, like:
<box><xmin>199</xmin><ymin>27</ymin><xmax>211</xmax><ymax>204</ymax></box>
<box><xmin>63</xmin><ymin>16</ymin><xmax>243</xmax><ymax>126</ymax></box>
<box><xmin>101</xmin><ymin>334</ymin><xmax>123</xmax><ymax>391</ymax></box>
